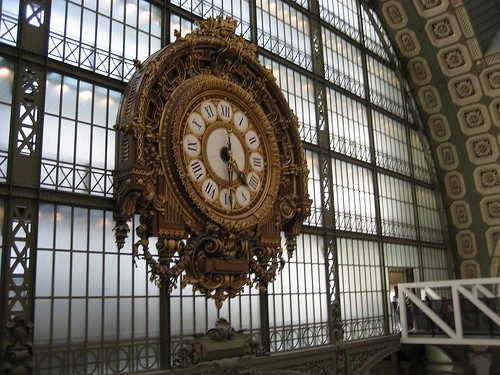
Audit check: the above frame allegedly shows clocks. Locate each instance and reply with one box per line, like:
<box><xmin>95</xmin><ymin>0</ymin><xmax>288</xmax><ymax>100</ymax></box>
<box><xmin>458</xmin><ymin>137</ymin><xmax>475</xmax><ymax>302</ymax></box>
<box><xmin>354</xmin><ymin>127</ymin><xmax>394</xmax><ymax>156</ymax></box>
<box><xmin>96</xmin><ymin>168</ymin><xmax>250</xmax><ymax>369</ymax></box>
<box><xmin>112</xmin><ymin>17</ymin><xmax>312</xmax><ymax>310</ymax></box>
